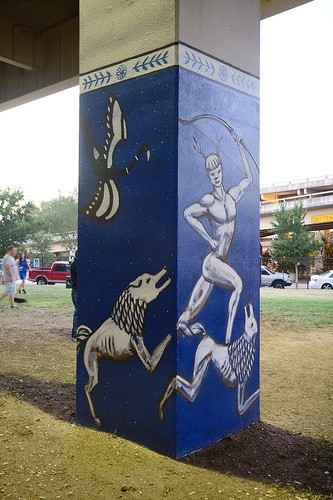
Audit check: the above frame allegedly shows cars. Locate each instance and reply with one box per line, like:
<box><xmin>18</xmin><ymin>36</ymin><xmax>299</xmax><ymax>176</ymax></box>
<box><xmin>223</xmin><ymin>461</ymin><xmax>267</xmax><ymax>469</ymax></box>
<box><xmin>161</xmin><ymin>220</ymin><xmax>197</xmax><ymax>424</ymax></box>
<box><xmin>308</xmin><ymin>269</ymin><xmax>333</xmax><ymax>290</ymax></box>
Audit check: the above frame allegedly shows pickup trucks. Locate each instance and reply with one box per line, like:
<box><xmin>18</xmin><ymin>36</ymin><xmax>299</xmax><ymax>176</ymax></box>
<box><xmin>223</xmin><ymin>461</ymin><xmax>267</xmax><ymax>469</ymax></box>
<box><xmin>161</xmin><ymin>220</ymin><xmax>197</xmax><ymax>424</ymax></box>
<box><xmin>27</xmin><ymin>261</ymin><xmax>72</xmax><ymax>285</ymax></box>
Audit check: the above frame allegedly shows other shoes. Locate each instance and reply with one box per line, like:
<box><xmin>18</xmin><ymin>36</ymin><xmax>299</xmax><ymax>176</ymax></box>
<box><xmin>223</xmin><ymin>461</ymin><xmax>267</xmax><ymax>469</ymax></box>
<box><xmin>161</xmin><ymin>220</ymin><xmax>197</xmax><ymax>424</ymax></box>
<box><xmin>10</xmin><ymin>304</ymin><xmax>17</xmax><ymax>308</ymax></box>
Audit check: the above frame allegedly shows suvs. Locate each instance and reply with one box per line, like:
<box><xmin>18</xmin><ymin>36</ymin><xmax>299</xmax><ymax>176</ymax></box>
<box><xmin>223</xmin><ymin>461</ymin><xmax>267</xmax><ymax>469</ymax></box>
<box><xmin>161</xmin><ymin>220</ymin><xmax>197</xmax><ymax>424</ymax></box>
<box><xmin>260</xmin><ymin>265</ymin><xmax>292</xmax><ymax>289</ymax></box>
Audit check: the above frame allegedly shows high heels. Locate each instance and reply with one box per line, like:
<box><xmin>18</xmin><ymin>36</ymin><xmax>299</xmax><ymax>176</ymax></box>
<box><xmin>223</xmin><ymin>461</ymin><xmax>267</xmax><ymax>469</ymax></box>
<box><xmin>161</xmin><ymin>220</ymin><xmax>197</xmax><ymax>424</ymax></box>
<box><xmin>22</xmin><ymin>289</ymin><xmax>26</xmax><ymax>294</ymax></box>
<box><xmin>19</xmin><ymin>288</ymin><xmax>22</xmax><ymax>293</ymax></box>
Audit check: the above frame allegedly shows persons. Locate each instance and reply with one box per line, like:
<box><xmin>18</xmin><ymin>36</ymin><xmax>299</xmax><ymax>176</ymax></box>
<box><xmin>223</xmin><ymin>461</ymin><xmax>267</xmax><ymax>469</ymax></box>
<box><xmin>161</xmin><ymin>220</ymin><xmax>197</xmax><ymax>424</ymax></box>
<box><xmin>0</xmin><ymin>245</ymin><xmax>32</xmax><ymax>309</ymax></box>
<box><xmin>70</xmin><ymin>250</ymin><xmax>77</xmax><ymax>342</ymax></box>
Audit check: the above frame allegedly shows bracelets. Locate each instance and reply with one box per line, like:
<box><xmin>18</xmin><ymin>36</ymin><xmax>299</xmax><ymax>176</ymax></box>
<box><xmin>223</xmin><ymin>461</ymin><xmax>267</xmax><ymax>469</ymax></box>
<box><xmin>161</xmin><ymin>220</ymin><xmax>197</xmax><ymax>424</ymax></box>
<box><xmin>28</xmin><ymin>266</ymin><xmax>30</xmax><ymax>268</ymax></box>
